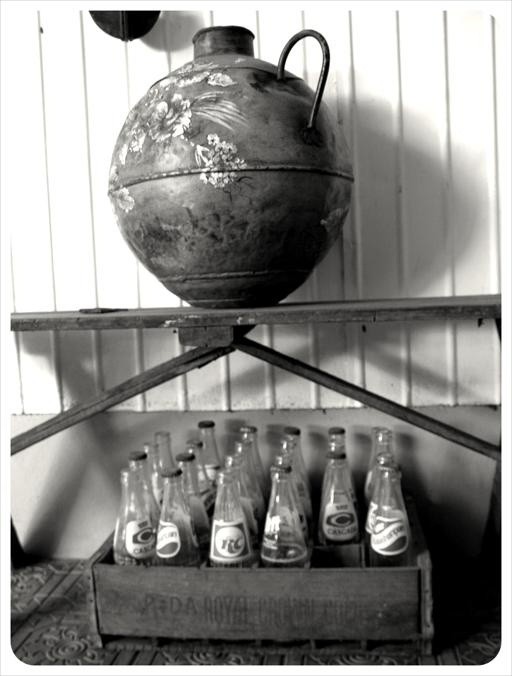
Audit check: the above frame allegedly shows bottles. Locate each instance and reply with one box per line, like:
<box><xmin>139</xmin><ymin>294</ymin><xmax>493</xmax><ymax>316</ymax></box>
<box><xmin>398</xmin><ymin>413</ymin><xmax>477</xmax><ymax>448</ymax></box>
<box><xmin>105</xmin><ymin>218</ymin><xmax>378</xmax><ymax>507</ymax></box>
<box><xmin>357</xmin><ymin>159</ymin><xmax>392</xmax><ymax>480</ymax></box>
<box><xmin>112</xmin><ymin>421</ymin><xmax>418</xmax><ymax>567</ymax></box>
